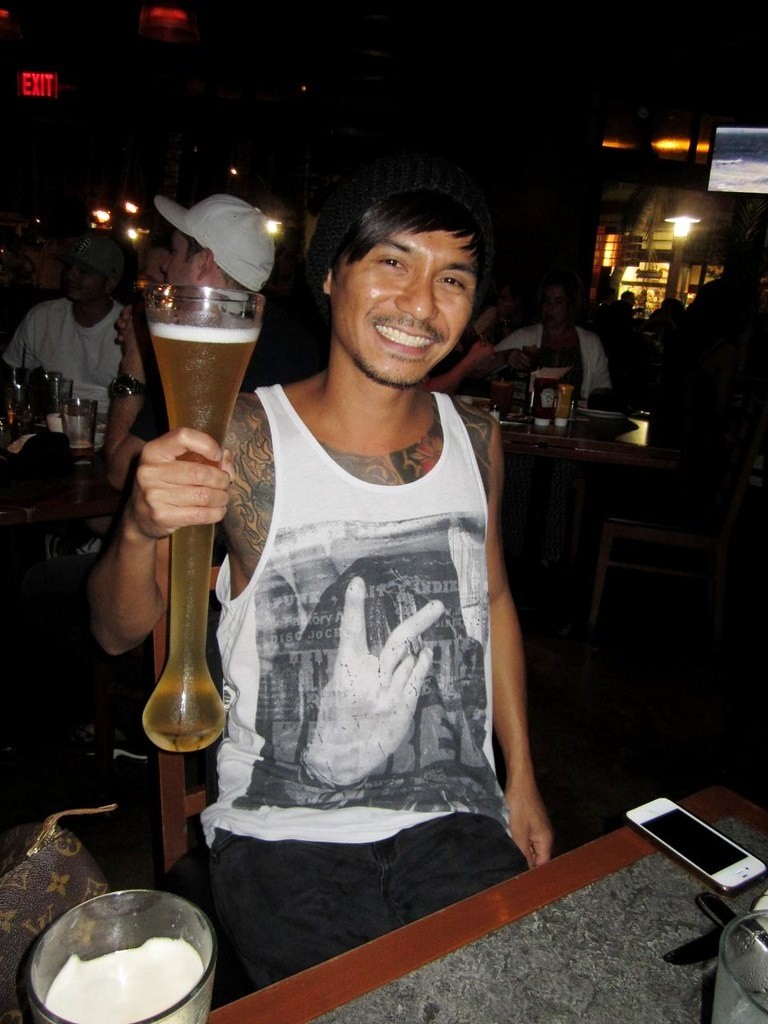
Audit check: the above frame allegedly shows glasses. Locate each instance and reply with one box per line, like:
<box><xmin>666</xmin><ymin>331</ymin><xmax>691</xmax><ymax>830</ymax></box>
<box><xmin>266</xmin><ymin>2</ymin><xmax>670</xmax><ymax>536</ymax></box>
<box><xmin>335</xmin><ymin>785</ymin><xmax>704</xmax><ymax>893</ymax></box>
<box><xmin>63</xmin><ymin>258</ymin><xmax>84</xmax><ymax>273</ymax></box>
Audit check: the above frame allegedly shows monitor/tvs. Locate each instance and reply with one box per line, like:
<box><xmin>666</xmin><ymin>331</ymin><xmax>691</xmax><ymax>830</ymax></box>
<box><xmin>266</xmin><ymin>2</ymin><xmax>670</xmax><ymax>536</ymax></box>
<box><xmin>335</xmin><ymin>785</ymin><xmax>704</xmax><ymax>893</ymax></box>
<box><xmin>705</xmin><ymin>123</ymin><xmax>768</xmax><ymax>196</ymax></box>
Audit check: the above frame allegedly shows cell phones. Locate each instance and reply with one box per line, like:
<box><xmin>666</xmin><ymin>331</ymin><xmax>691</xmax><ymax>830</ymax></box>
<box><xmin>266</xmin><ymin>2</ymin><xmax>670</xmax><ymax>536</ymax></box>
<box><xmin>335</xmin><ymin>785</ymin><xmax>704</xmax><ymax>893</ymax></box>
<box><xmin>625</xmin><ymin>795</ymin><xmax>768</xmax><ymax>897</ymax></box>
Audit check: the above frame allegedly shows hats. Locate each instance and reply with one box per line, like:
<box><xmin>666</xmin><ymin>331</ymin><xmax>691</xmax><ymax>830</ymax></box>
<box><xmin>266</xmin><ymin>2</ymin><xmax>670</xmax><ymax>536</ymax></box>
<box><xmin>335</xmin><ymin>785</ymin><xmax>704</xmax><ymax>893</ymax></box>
<box><xmin>48</xmin><ymin>235</ymin><xmax>125</xmax><ymax>282</ymax></box>
<box><xmin>154</xmin><ymin>194</ymin><xmax>276</xmax><ymax>291</ymax></box>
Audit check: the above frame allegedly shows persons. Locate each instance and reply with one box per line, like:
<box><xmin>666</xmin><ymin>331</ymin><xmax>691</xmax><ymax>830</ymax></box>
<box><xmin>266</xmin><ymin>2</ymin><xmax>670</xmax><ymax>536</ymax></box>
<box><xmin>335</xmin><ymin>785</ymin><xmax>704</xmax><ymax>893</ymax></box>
<box><xmin>88</xmin><ymin>163</ymin><xmax>553</xmax><ymax>1007</ymax></box>
<box><xmin>0</xmin><ymin>194</ymin><xmax>768</xmax><ymax>660</ymax></box>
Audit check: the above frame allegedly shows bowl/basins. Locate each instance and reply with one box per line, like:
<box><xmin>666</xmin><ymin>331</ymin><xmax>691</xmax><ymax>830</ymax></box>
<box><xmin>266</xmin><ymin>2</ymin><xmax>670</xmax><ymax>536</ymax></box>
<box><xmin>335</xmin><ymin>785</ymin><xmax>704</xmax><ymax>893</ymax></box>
<box><xmin>45</xmin><ymin>413</ymin><xmax>64</xmax><ymax>434</ymax></box>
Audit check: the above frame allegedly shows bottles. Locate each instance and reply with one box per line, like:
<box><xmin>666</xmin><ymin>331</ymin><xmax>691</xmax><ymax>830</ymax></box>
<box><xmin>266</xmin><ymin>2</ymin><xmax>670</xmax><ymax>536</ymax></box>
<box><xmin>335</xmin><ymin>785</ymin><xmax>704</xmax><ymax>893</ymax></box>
<box><xmin>534</xmin><ymin>377</ymin><xmax>574</xmax><ymax>428</ymax></box>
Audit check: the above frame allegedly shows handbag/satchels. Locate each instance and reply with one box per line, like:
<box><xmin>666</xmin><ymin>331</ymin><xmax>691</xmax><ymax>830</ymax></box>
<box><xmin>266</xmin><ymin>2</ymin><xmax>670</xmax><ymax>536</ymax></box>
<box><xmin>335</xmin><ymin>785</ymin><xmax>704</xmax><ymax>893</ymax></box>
<box><xmin>0</xmin><ymin>803</ymin><xmax>123</xmax><ymax>1024</ymax></box>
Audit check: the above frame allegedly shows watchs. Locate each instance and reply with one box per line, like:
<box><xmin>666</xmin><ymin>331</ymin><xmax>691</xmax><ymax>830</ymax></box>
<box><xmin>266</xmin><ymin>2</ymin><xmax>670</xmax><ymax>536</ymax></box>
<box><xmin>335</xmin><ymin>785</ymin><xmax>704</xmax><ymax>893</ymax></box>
<box><xmin>108</xmin><ymin>367</ymin><xmax>150</xmax><ymax>397</ymax></box>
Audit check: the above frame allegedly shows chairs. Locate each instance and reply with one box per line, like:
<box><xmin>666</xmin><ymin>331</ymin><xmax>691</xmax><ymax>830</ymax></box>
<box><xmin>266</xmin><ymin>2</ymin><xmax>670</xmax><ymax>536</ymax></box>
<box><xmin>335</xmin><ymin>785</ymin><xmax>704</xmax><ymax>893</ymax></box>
<box><xmin>154</xmin><ymin>565</ymin><xmax>210</xmax><ymax>911</ymax></box>
<box><xmin>576</xmin><ymin>377</ymin><xmax>768</xmax><ymax>678</ymax></box>
<box><xmin>92</xmin><ymin>641</ymin><xmax>154</xmax><ymax>809</ymax></box>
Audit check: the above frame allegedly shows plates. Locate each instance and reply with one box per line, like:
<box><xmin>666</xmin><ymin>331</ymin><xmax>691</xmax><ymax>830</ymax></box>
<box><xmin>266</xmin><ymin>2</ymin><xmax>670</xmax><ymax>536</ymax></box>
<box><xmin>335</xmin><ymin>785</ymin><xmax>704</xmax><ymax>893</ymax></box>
<box><xmin>577</xmin><ymin>408</ymin><xmax>626</xmax><ymax>419</ymax></box>
<box><xmin>7</xmin><ymin>433</ymin><xmax>36</xmax><ymax>455</ymax></box>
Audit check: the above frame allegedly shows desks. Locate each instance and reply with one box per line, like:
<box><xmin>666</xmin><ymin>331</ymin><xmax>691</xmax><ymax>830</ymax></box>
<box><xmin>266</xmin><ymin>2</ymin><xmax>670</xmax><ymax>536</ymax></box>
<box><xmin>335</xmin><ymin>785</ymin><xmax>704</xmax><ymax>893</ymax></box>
<box><xmin>0</xmin><ymin>450</ymin><xmax>136</xmax><ymax>526</ymax></box>
<box><xmin>206</xmin><ymin>785</ymin><xmax>767</xmax><ymax>1024</ymax></box>
<box><xmin>497</xmin><ymin>416</ymin><xmax>681</xmax><ymax>472</ymax></box>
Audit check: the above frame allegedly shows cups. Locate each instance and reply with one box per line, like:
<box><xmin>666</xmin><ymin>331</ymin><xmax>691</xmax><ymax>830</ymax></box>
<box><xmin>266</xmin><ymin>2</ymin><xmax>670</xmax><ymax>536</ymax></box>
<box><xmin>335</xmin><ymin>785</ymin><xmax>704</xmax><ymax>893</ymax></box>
<box><xmin>710</xmin><ymin>909</ymin><xmax>768</xmax><ymax>1024</ymax></box>
<box><xmin>490</xmin><ymin>379</ymin><xmax>514</xmax><ymax>411</ymax></box>
<box><xmin>142</xmin><ymin>284</ymin><xmax>264</xmax><ymax>751</ymax></box>
<box><xmin>0</xmin><ymin>371</ymin><xmax>98</xmax><ymax>464</ymax></box>
<box><xmin>25</xmin><ymin>888</ymin><xmax>218</xmax><ymax>1024</ymax></box>
